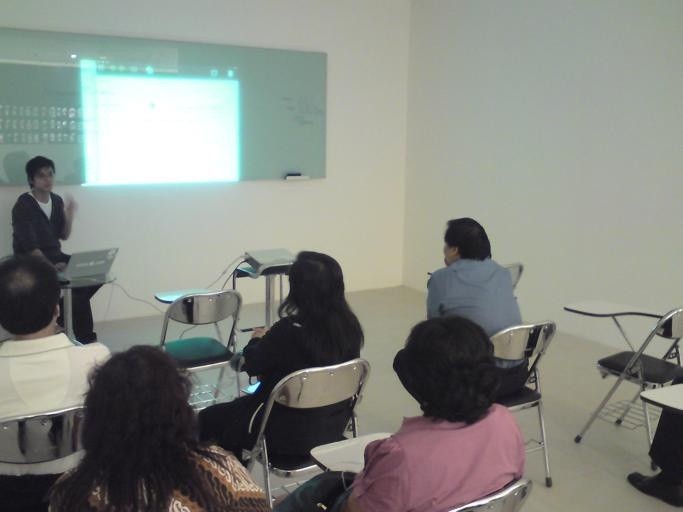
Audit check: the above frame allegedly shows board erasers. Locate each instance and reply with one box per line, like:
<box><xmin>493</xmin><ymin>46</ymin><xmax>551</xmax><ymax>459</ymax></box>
<box><xmin>285</xmin><ymin>173</ymin><xmax>306</xmax><ymax>180</ymax></box>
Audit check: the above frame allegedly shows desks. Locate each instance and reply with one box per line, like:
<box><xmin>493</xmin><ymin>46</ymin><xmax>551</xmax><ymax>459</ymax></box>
<box><xmin>309</xmin><ymin>432</ymin><xmax>392</xmax><ymax>475</ymax></box>
<box><xmin>154</xmin><ymin>290</ymin><xmax>209</xmax><ymax>303</ymax></box>
<box><xmin>563</xmin><ymin>305</ymin><xmax>671</xmax><ymax>318</ymax></box>
<box><xmin>640</xmin><ymin>384</ymin><xmax>683</xmax><ymax>411</ymax></box>
<box><xmin>58</xmin><ymin>271</ymin><xmax>119</xmax><ymax>345</ymax></box>
<box><xmin>231</xmin><ymin>266</ymin><xmax>294</xmax><ymax>346</ymax></box>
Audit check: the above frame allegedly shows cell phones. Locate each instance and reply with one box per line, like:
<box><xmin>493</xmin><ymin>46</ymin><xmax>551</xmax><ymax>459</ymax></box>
<box><xmin>58</xmin><ymin>277</ymin><xmax>72</xmax><ymax>285</ymax></box>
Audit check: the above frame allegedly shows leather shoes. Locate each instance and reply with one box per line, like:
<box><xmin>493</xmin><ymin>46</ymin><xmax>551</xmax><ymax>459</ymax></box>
<box><xmin>624</xmin><ymin>468</ymin><xmax>683</xmax><ymax>508</ymax></box>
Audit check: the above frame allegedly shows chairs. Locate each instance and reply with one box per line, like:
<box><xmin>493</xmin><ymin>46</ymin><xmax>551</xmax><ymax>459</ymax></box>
<box><xmin>452</xmin><ymin>472</ymin><xmax>532</xmax><ymax>512</ymax></box>
<box><xmin>236</xmin><ymin>358</ymin><xmax>371</xmax><ymax>511</ymax></box>
<box><xmin>0</xmin><ymin>406</ymin><xmax>93</xmax><ymax>493</ymax></box>
<box><xmin>156</xmin><ymin>291</ymin><xmax>241</xmax><ymax>412</ymax></box>
<box><xmin>503</xmin><ymin>262</ymin><xmax>524</xmax><ymax>287</ymax></box>
<box><xmin>488</xmin><ymin>320</ymin><xmax>556</xmax><ymax>487</ymax></box>
<box><xmin>574</xmin><ymin>307</ymin><xmax>683</xmax><ymax>470</ymax></box>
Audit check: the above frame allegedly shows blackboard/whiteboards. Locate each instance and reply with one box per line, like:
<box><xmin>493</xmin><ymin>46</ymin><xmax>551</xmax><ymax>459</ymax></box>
<box><xmin>1</xmin><ymin>28</ymin><xmax>328</xmax><ymax>188</ymax></box>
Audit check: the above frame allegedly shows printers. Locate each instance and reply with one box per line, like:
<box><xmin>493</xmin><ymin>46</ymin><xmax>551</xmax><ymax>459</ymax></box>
<box><xmin>245</xmin><ymin>246</ymin><xmax>295</xmax><ymax>273</ymax></box>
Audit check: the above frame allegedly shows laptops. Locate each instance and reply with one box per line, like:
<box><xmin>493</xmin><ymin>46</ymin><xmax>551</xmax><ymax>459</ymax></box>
<box><xmin>53</xmin><ymin>245</ymin><xmax>119</xmax><ymax>278</ymax></box>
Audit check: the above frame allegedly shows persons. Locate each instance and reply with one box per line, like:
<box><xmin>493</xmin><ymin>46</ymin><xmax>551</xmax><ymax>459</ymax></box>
<box><xmin>0</xmin><ymin>254</ymin><xmax>111</xmax><ymax>508</ymax></box>
<box><xmin>272</xmin><ymin>315</ymin><xmax>525</xmax><ymax>512</ymax></box>
<box><xmin>11</xmin><ymin>156</ymin><xmax>104</xmax><ymax>342</ymax></box>
<box><xmin>43</xmin><ymin>344</ymin><xmax>271</xmax><ymax>511</ymax></box>
<box><xmin>198</xmin><ymin>251</ymin><xmax>364</xmax><ymax>470</ymax></box>
<box><xmin>427</xmin><ymin>218</ymin><xmax>529</xmax><ymax>399</ymax></box>
<box><xmin>628</xmin><ymin>376</ymin><xmax>682</xmax><ymax>509</ymax></box>
